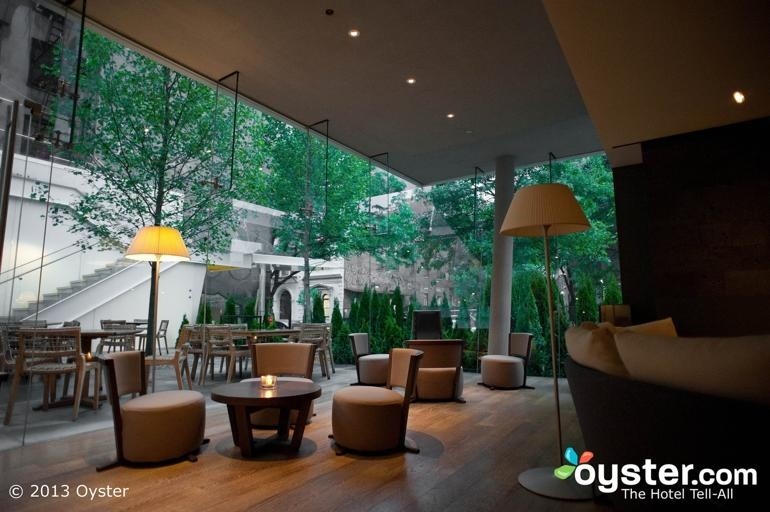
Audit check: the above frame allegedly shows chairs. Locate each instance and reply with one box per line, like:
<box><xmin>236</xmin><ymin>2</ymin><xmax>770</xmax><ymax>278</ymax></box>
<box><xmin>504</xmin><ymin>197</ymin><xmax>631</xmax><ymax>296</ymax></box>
<box><xmin>134</xmin><ymin>320</ymin><xmax>169</xmax><ymax>356</ymax></box>
<box><xmin>95</xmin><ymin>320</ymin><xmax>126</xmax><ymax>354</ymax></box>
<box><xmin>181</xmin><ymin>321</ymin><xmax>250</xmax><ymax>387</ymax></box>
<box><xmin>1</xmin><ymin>320</ymin><xmax>94</xmax><ymax>422</ymax></box>
<box><xmin>292</xmin><ymin>322</ymin><xmax>336</xmax><ymax>380</ymax></box>
<box><xmin>143</xmin><ymin>324</ymin><xmax>195</xmax><ymax>390</ymax></box>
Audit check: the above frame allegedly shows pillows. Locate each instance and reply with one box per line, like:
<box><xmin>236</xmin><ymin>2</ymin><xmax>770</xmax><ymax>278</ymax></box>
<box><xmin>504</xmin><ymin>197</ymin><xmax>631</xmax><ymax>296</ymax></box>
<box><xmin>612</xmin><ymin>328</ymin><xmax>768</xmax><ymax>402</ymax></box>
<box><xmin>563</xmin><ymin>325</ymin><xmax>623</xmax><ymax>378</ymax></box>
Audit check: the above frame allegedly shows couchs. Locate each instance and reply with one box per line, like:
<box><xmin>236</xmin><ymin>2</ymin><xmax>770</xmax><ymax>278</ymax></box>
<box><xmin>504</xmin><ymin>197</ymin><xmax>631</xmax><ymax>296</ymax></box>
<box><xmin>327</xmin><ymin>348</ymin><xmax>426</xmax><ymax>457</ymax></box>
<box><xmin>564</xmin><ymin>355</ymin><xmax>770</xmax><ymax>511</ymax></box>
<box><xmin>410</xmin><ymin>309</ymin><xmax>442</xmax><ymax>340</ymax></box>
<box><xmin>477</xmin><ymin>332</ymin><xmax>536</xmax><ymax>391</ymax></box>
<box><xmin>402</xmin><ymin>339</ymin><xmax>466</xmax><ymax>405</ymax></box>
<box><xmin>349</xmin><ymin>332</ymin><xmax>390</xmax><ymax>386</ymax></box>
<box><xmin>96</xmin><ymin>351</ymin><xmax>211</xmax><ymax>473</ymax></box>
<box><xmin>240</xmin><ymin>340</ymin><xmax>317</xmax><ymax>431</ymax></box>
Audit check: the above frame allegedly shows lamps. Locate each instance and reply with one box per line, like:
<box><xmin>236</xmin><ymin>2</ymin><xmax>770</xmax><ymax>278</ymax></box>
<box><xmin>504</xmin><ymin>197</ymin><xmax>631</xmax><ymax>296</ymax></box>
<box><xmin>124</xmin><ymin>225</ymin><xmax>192</xmax><ymax>394</ymax></box>
<box><xmin>498</xmin><ymin>182</ymin><xmax>593</xmax><ymax>503</ymax></box>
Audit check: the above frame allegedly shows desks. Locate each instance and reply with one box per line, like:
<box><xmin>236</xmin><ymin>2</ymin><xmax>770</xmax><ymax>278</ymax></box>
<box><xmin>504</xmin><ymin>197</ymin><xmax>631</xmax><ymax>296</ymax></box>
<box><xmin>210</xmin><ymin>381</ymin><xmax>322</xmax><ymax>460</ymax></box>
<box><xmin>230</xmin><ymin>327</ymin><xmax>300</xmax><ymax>370</ymax></box>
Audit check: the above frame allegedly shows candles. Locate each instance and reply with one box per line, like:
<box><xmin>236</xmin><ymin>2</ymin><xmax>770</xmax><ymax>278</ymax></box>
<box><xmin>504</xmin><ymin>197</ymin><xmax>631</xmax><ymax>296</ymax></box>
<box><xmin>265</xmin><ymin>378</ymin><xmax>273</xmax><ymax>385</ymax></box>
